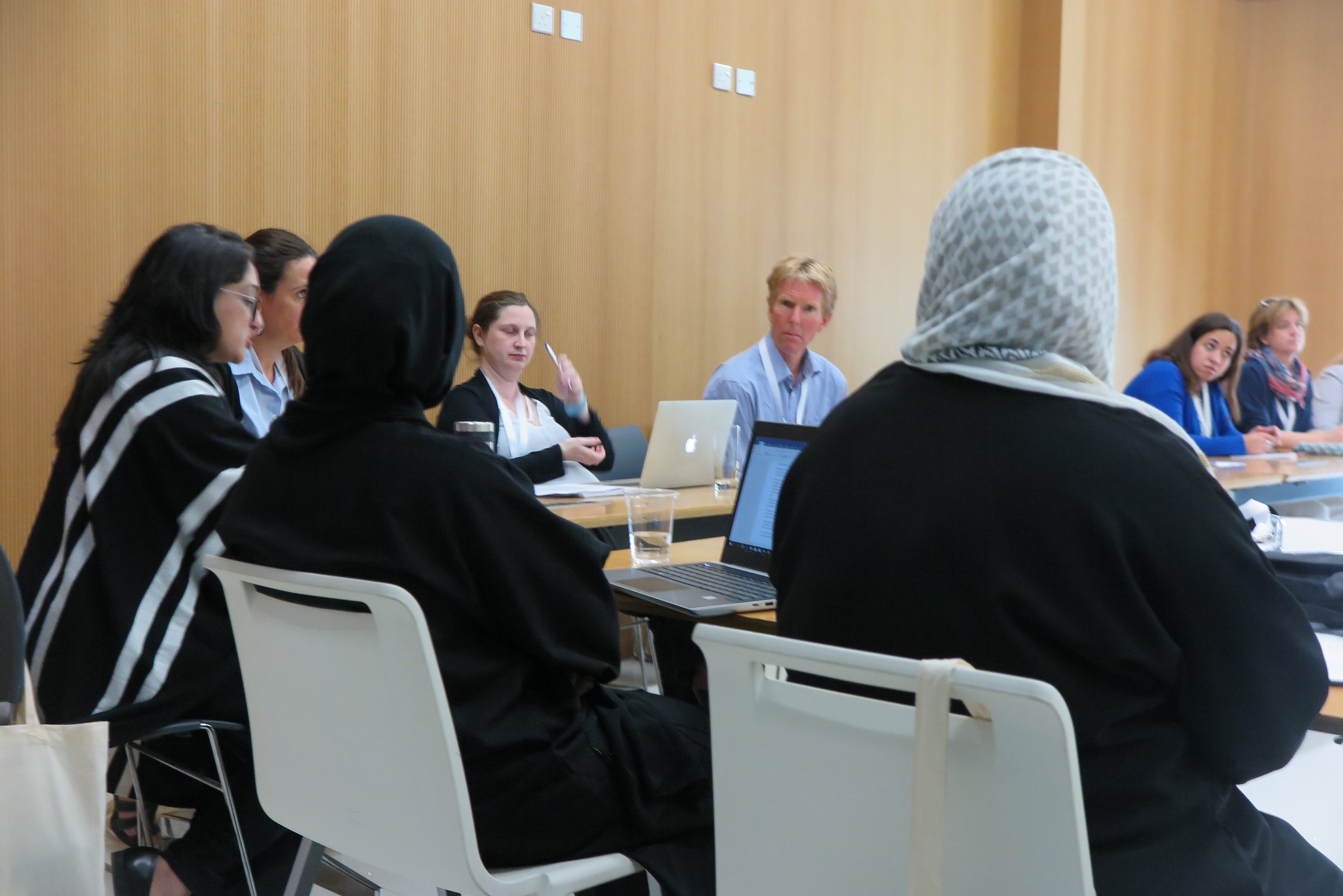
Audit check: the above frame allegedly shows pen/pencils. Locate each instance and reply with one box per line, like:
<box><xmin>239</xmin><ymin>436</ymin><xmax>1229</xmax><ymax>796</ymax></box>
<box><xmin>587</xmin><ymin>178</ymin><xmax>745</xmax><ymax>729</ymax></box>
<box><xmin>544</xmin><ymin>342</ymin><xmax>571</xmax><ymax>391</ymax></box>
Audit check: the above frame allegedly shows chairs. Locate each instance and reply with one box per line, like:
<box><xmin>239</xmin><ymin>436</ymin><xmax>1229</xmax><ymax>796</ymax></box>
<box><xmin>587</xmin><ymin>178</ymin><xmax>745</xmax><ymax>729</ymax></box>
<box><xmin>690</xmin><ymin>623</ymin><xmax>1099</xmax><ymax>895</ymax></box>
<box><xmin>201</xmin><ymin>553</ymin><xmax>649</xmax><ymax>896</ymax></box>
<box><xmin>585</xmin><ymin>424</ymin><xmax>663</xmax><ymax>697</ymax></box>
<box><xmin>0</xmin><ymin>545</ymin><xmax>262</xmax><ymax>896</ymax></box>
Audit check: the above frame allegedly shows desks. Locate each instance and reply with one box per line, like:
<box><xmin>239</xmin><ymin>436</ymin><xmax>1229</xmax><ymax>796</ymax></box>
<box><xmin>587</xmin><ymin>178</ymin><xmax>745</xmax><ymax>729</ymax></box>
<box><xmin>534</xmin><ymin>451</ymin><xmax>1343</xmax><ymax>736</ymax></box>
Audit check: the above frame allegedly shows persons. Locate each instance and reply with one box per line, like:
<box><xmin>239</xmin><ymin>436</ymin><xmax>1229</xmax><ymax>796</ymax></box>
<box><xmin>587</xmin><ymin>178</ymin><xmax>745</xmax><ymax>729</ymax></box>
<box><xmin>14</xmin><ymin>223</ymin><xmax>316</xmax><ymax>896</ymax></box>
<box><xmin>217</xmin><ymin>214</ymin><xmax>715</xmax><ymax>896</ymax></box>
<box><xmin>1236</xmin><ymin>297</ymin><xmax>1342</xmax><ymax>451</ymax></box>
<box><xmin>771</xmin><ymin>148</ymin><xmax>1342</xmax><ymax>895</ymax></box>
<box><xmin>704</xmin><ymin>256</ymin><xmax>848</xmax><ymax>479</ymax></box>
<box><xmin>435</xmin><ymin>289</ymin><xmax>729</xmax><ymax>709</ymax></box>
<box><xmin>1122</xmin><ymin>312</ymin><xmax>1284</xmax><ymax>456</ymax></box>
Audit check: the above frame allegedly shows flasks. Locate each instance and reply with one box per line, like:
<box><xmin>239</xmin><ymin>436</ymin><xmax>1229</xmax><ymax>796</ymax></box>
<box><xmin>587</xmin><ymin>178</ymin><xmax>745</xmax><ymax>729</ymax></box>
<box><xmin>454</xmin><ymin>420</ymin><xmax>495</xmax><ymax>453</ymax></box>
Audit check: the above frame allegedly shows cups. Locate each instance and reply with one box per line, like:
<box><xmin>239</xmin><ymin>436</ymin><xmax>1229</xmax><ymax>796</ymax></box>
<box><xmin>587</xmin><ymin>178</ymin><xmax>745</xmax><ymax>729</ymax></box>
<box><xmin>713</xmin><ymin>425</ymin><xmax>740</xmax><ymax>491</ymax></box>
<box><xmin>623</xmin><ymin>488</ymin><xmax>678</xmax><ymax>566</ymax></box>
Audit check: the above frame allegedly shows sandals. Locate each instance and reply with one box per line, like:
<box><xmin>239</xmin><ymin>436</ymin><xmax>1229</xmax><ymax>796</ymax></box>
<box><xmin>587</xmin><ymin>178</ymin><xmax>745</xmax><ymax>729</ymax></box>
<box><xmin>109</xmin><ymin>795</ymin><xmax>163</xmax><ymax>850</ymax></box>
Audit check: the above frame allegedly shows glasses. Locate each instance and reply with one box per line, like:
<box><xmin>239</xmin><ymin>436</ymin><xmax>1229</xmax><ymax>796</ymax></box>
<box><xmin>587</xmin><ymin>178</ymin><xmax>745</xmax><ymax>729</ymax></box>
<box><xmin>1259</xmin><ymin>297</ymin><xmax>1280</xmax><ymax>308</ymax></box>
<box><xmin>219</xmin><ymin>287</ymin><xmax>262</xmax><ymax>320</ymax></box>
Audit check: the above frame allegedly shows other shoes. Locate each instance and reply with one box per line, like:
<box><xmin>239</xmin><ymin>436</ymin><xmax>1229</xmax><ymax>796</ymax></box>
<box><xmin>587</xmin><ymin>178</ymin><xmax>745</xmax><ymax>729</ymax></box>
<box><xmin>111</xmin><ymin>845</ymin><xmax>158</xmax><ymax>896</ymax></box>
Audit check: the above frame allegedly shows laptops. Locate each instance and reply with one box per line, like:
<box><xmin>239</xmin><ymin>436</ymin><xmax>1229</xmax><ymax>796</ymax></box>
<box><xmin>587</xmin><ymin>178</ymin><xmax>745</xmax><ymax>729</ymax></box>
<box><xmin>601</xmin><ymin>418</ymin><xmax>857</xmax><ymax>621</ymax></box>
<box><xmin>587</xmin><ymin>398</ymin><xmax>742</xmax><ymax>491</ymax></box>
